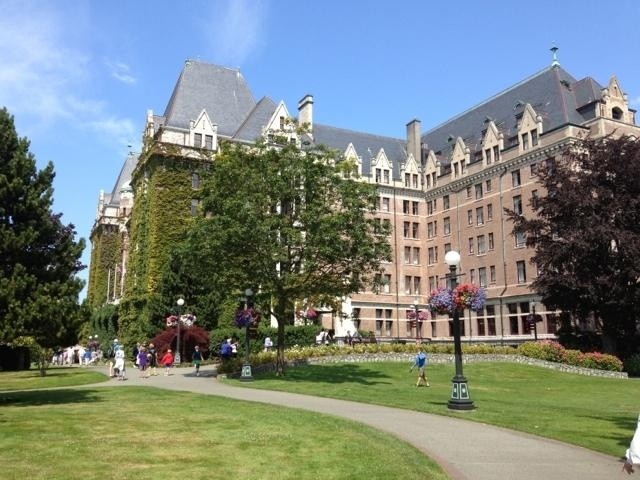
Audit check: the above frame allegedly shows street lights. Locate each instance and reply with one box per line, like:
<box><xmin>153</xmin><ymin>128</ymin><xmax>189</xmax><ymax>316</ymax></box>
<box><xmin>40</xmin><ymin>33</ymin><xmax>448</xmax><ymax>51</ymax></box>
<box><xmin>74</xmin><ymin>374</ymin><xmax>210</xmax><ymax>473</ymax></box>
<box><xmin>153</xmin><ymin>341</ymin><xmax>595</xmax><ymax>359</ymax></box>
<box><xmin>173</xmin><ymin>298</ymin><xmax>185</xmax><ymax>366</ymax></box>
<box><xmin>414</xmin><ymin>300</ymin><xmax>420</xmax><ymax>339</ymax></box>
<box><xmin>531</xmin><ymin>299</ymin><xmax>538</xmax><ymax>341</ymax></box>
<box><xmin>240</xmin><ymin>284</ymin><xmax>254</xmax><ymax>382</ymax></box>
<box><xmin>444</xmin><ymin>250</ymin><xmax>475</xmax><ymax>410</ymax></box>
<box><xmin>89</xmin><ymin>332</ymin><xmax>99</xmax><ymax>362</ymax></box>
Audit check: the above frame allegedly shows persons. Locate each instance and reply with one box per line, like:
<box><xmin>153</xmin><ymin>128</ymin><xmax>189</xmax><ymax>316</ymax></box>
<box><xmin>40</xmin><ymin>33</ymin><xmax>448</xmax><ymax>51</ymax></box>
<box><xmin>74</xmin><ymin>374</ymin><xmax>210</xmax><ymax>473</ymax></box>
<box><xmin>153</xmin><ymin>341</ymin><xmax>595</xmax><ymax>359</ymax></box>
<box><xmin>265</xmin><ymin>335</ymin><xmax>273</xmax><ymax>352</ymax></box>
<box><xmin>409</xmin><ymin>347</ymin><xmax>430</xmax><ymax>390</ymax></box>
<box><xmin>622</xmin><ymin>413</ymin><xmax>640</xmax><ymax>476</ymax></box>
<box><xmin>316</xmin><ymin>328</ymin><xmax>331</xmax><ymax>347</ymax></box>
<box><xmin>344</xmin><ymin>331</ymin><xmax>352</xmax><ymax>346</ymax></box>
<box><xmin>191</xmin><ymin>345</ymin><xmax>204</xmax><ymax>377</ymax></box>
<box><xmin>52</xmin><ymin>339</ymin><xmax>173</xmax><ymax>381</ymax></box>
<box><xmin>220</xmin><ymin>332</ymin><xmax>242</xmax><ymax>364</ymax></box>
